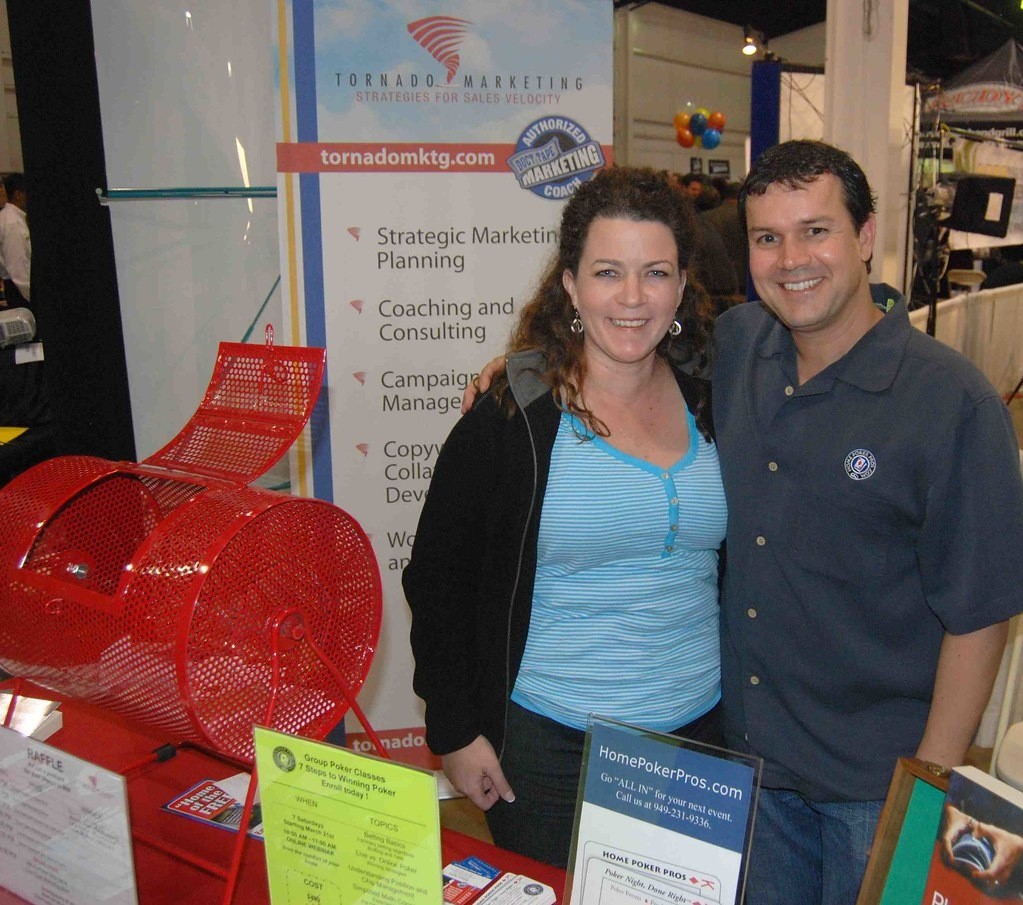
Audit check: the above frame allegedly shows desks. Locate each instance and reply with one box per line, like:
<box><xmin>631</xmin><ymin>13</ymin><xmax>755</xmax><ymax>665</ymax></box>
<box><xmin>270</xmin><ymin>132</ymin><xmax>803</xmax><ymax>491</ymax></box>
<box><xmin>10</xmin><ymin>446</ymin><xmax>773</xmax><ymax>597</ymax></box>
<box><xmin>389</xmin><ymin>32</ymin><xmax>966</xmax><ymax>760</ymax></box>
<box><xmin>0</xmin><ymin>675</ymin><xmax>573</xmax><ymax>905</ymax></box>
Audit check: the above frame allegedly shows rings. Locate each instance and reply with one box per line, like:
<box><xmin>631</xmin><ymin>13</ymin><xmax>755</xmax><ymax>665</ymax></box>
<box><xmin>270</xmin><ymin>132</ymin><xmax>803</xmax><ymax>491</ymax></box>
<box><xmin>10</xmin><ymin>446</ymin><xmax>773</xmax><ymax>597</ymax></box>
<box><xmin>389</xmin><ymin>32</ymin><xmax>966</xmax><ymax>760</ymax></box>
<box><xmin>995</xmin><ymin>880</ymin><xmax>1000</xmax><ymax>887</ymax></box>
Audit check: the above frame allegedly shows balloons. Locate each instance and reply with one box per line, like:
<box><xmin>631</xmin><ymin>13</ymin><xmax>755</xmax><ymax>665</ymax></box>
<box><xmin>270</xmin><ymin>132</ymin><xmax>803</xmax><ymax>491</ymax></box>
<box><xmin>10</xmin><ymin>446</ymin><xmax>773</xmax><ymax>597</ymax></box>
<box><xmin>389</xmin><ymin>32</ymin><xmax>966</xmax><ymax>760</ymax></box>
<box><xmin>675</xmin><ymin>129</ymin><xmax>694</xmax><ymax>148</ymax></box>
<box><xmin>695</xmin><ymin>135</ymin><xmax>704</xmax><ymax>149</ymax></box>
<box><xmin>689</xmin><ymin>114</ymin><xmax>707</xmax><ymax>136</ymax></box>
<box><xmin>694</xmin><ymin>107</ymin><xmax>710</xmax><ymax>120</ymax></box>
<box><xmin>672</xmin><ymin>112</ymin><xmax>691</xmax><ymax>131</ymax></box>
<box><xmin>702</xmin><ymin>128</ymin><xmax>721</xmax><ymax>150</ymax></box>
<box><xmin>708</xmin><ymin>112</ymin><xmax>726</xmax><ymax>131</ymax></box>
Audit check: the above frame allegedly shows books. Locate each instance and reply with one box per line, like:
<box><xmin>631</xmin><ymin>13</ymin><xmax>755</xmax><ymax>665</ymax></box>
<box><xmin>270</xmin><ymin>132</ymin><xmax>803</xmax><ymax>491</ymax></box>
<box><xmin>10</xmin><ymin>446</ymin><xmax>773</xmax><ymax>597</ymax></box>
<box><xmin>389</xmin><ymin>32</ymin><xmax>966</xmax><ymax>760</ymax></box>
<box><xmin>922</xmin><ymin>765</ymin><xmax>1023</xmax><ymax>905</ymax></box>
<box><xmin>0</xmin><ymin>692</ymin><xmax>63</xmax><ymax>742</ymax></box>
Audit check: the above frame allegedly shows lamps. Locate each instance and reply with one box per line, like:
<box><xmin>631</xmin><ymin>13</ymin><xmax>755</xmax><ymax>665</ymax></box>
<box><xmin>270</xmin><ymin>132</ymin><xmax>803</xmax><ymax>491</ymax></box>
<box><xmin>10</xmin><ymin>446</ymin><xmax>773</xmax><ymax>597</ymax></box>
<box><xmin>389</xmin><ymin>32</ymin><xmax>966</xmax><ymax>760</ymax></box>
<box><xmin>743</xmin><ymin>27</ymin><xmax>757</xmax><ymax>55</ymax></box>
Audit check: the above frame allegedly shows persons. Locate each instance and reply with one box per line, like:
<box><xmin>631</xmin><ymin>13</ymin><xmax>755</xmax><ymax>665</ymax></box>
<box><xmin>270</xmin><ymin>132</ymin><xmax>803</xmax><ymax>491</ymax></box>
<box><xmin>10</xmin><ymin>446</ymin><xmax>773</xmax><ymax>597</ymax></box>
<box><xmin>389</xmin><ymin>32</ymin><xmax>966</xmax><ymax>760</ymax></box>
<box><xmin>936</xmin><ymin>769</ymin><xmax>1023</xmax><ymax>896</ymax></box>
<box><xmin>671</xmin><ymin>173</ymin><xmax>749</xmax><ymax>296</ymax></box>
<box><xmin>461</xmin><ymin>140</ymin><xmax>1023</xmax><ymax>905</ymax></box>
<box><xmin>402</xmin><ymin>166</ymin><xmax>728</xmax><ymax>872</ymax></box>
<box><xmin>0</xmin><ymin>173</ymin><xmax>31</xmax><ymax>314</ymax></box>
